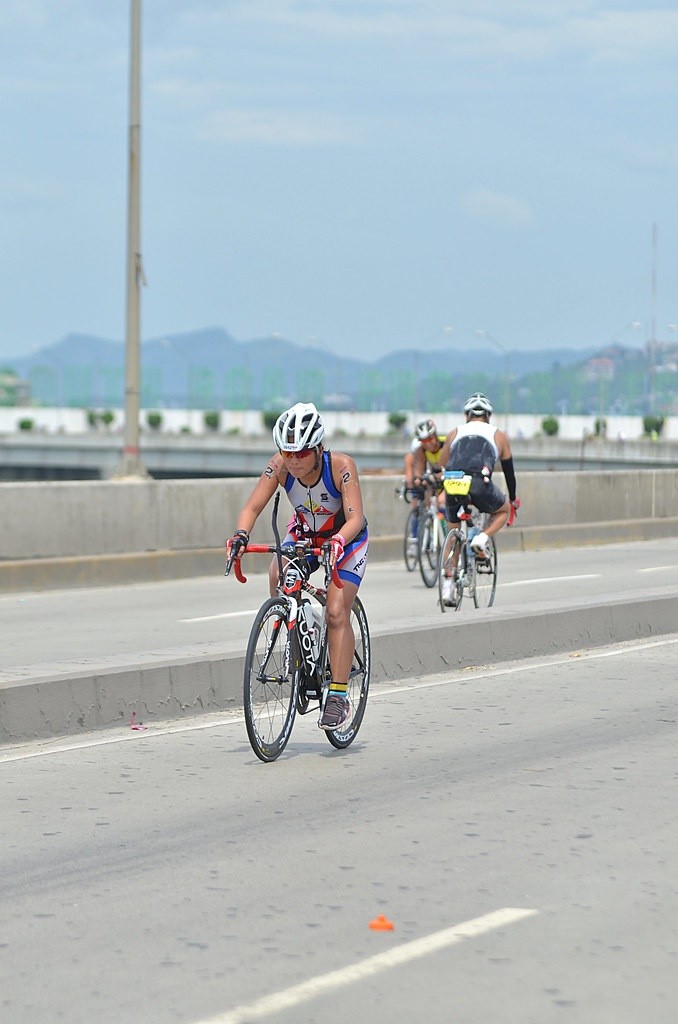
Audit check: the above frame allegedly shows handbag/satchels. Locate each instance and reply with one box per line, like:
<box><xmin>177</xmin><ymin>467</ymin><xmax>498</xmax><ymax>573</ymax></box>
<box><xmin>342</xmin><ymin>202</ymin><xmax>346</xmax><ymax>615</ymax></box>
<box><xmin>442</xmin><ymin>469</ymin><xmax>474</xmax><ymax>496</ymax></box>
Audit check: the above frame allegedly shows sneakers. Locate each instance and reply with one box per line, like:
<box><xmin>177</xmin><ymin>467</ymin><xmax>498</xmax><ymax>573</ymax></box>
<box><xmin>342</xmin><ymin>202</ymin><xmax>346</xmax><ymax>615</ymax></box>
<box><xmin>318</xmin><ymin>696</ymin><xmax>352</xmax><ymax>728</ymax></box>
<box><xmin>471</xmin><ymin>535</ymin><xmax>493</xmax><ymax>561</ymax></box>
<box><xmin>406</xmin><ymin>538</ymin><xmax>419</xmax><ymax>556</ymax></box>
<box><xmin>441</xmin><ymin>578</ymin><xmax>456</xmax><ymax>603</ymax></box>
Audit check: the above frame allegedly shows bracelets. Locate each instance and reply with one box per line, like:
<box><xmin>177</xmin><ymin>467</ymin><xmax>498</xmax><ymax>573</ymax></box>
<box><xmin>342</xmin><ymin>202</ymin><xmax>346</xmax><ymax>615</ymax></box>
<box><xmin>331</xmin><ymin>533</ymin><xmax>346</xmax><ymax>547</ymax></box>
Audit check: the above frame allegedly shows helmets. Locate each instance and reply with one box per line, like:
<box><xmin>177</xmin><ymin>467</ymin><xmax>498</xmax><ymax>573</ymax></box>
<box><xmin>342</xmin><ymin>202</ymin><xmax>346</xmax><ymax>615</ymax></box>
<box><xmin>274</xmin><ymin>402</ymin><xmax>324</xmax><ymax>451</ymax></box>
<box><xmin>415</xmin><ymin>420</ymin><xmax>437</xmax><ymax>439</ymax></box>
<box><xmin>464</xmin><ymin>392</ymin><xmax>493</xmax><ymax>415</ymax></box>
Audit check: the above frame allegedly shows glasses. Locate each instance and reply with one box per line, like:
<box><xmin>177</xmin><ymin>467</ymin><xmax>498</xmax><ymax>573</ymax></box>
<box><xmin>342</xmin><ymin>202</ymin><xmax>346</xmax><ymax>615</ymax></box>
<box><xmin>420</xmin><ymin>435</ymin><xmax>437</xmax><ymax>443</ymax></box>
<box><xmin>278</xmin><ymin>450</ymin><xmax>312</xmax><ymax>459</ymax></box>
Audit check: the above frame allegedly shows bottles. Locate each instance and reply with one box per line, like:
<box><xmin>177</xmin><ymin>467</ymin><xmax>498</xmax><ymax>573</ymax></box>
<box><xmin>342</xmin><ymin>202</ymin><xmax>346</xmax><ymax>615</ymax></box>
<box><xmin>309</xmin><ymin>628</ymin><xmax>319</xmax><ymax>659</ymax></box>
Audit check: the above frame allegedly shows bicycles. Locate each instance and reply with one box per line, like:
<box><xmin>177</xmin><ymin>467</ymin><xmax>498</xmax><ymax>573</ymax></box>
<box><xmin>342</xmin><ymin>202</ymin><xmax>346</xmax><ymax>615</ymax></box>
<box><xmin>410</xmin><ymin>474</ymin><xmax>459</xmax><ymax>587</ymax></box>
<box><xmin>223</xmin><ymin>536</ymin><xmax>371</xmax><ymax>763</ymax></box>
<box><xmin>394</xmin><ymin>475</ymin><xmax>442</xmax><ymax>572</ymax></box>
<box><xmin>437</xmin><ymin>489</ymin><xmax>518</xmax><ymax>614</ymax></box>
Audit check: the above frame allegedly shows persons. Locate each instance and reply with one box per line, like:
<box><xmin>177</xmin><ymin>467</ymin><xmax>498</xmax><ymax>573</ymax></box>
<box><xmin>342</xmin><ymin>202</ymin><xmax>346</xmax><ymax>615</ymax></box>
<box><xmin>224</xmin><ymin>401</ymin><xmax>373</xmax><ymax>732</ymax></box>
<box><xmin>404</xmin><ymin>437</ymin><xmax>428</xmax><ymax>557</ymax></box>
<box><xmin>413</xmin><ymin>419</ymin><xmax>447</xmax><ymax>547</ymax></box>
<box><xmin>438</xmin><ymin>391</ymin><xmax>521</xmax><ymax>608</ymax></box>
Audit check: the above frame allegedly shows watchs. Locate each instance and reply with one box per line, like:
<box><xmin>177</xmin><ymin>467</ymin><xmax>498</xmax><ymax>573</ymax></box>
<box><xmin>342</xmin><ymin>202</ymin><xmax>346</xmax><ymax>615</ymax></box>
<box><xmin>232</xmin><ymin>529</ymin><xmax>250</xmax><ymax>543</ymax></box>
